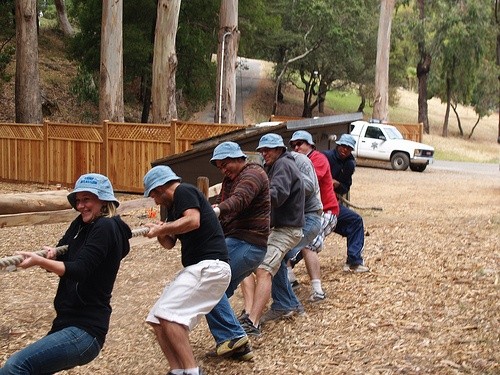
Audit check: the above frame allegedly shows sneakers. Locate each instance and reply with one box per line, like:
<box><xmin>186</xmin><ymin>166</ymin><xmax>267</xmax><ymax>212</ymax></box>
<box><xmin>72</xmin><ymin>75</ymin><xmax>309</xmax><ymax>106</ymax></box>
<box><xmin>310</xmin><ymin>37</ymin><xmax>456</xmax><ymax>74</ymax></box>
<box><xmin>263</xmin><ymin>305</ymin><xmax>304</xmax><ymax>321</ymax></box>
<box><xmin>343</xmin><ymin>265</ymin><xmax>369</xmax><ymax>272</ymax></box>
<box><xmin>238</xmin><ymin>309</ymin><xmax>250</xmax><ymax>323</ymax></box>
<box><xmin>207</xmin><ymin>336</ymin><xmax>248</xmax><ymax>357</ymax></box>
<box><xmin>229</xmin><ymin>345</ymin><xmax>254</xmax><ymax>359</ymax></box>
<box><xmin>290</xmin><ymin>280</ymin><xmax>301</xmax><ymax>290</ymax></box>
<box><xmin>239</xmin><ymin>318</ymin><xmax>260</xmax><ymax>335</ymax></box>
<box><xmin>305</xmin><ymin>292</ymin><xmax>326</xmax><ymax>302</ymax></box>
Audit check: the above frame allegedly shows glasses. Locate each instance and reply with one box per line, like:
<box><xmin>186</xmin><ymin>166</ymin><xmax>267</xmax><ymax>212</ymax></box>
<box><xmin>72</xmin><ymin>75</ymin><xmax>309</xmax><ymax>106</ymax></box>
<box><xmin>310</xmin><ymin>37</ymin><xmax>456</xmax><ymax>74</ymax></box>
<box><xmin>291</xmin><ymin>140</ymin><xmax>305</xmax><ymax>149</ymax></box>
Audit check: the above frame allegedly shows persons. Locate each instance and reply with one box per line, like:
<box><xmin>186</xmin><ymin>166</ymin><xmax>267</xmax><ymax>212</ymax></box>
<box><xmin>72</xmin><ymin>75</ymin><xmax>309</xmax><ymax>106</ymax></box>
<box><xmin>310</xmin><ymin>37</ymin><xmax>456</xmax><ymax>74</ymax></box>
<box><xmin>322</xmin><ymin>133</ymin><xmax>369</xmax><ymax>272</ymax></box>
<box><xmin>204</xmin><ymin>142</ymin><xmax>271</xmax><ymax>361</ymax></box>
<box><xmin>238</xmin><ymin>133</ymin><xmax>305</xmax><ymax>336</ymax></box>
<box><xmin>143</xmin><ymin>164</ymin><xmax>232</xmax><ymax>375</ymax></box>
<box><xmin>262</xmin><ymin>151</ymin><xmax>323</xmax><ymax>324</ymax></box>
<box><xmin>286</xmin><ymin>130</ymin><xmax>340</xmax><ymax>301</ymax></box>
<box><xmin>0</xmin><ymin>173</ymin><xmax>132</xmax><ymax>375</ymax></box>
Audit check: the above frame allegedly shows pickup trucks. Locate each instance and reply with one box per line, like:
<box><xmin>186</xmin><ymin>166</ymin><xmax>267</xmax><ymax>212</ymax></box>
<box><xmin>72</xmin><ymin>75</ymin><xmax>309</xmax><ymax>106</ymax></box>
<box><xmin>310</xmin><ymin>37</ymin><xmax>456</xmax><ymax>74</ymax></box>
<box><xmin>351</xmin><ymin>119</ymin><xmax>436</xmax><ymax>172</ymax></box>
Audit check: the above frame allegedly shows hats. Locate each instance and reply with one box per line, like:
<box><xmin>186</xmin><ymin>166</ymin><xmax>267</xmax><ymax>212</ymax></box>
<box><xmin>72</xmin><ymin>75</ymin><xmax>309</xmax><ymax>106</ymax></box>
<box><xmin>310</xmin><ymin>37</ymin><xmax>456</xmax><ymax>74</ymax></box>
<box><xmin>210</xmin><ymin>141</ymin><xmax>247</xmax><ymax>167</ymax></box>
<box><xmin>288</xmin><ymin>130</ymin><xmax>316</xmax><ymax>149</ymax></box>
<box><xmin>143</xmin><ymin>165</ymin><xmax>182</xmax><ymax>197</ymax></box>
<box><xmin>67</xmin><ymin>173</ymin><xmax>119</xmax><ymax>212</ymax></box>
<box><xmin>256</xmin><ymin>133</ymin><xmax>287</xmax><ymax>153</ymax></box>
<box><xmin>335</xmin><ymin>133</ymin><xmax>356</xmax><ymax>150</ymax></box>
